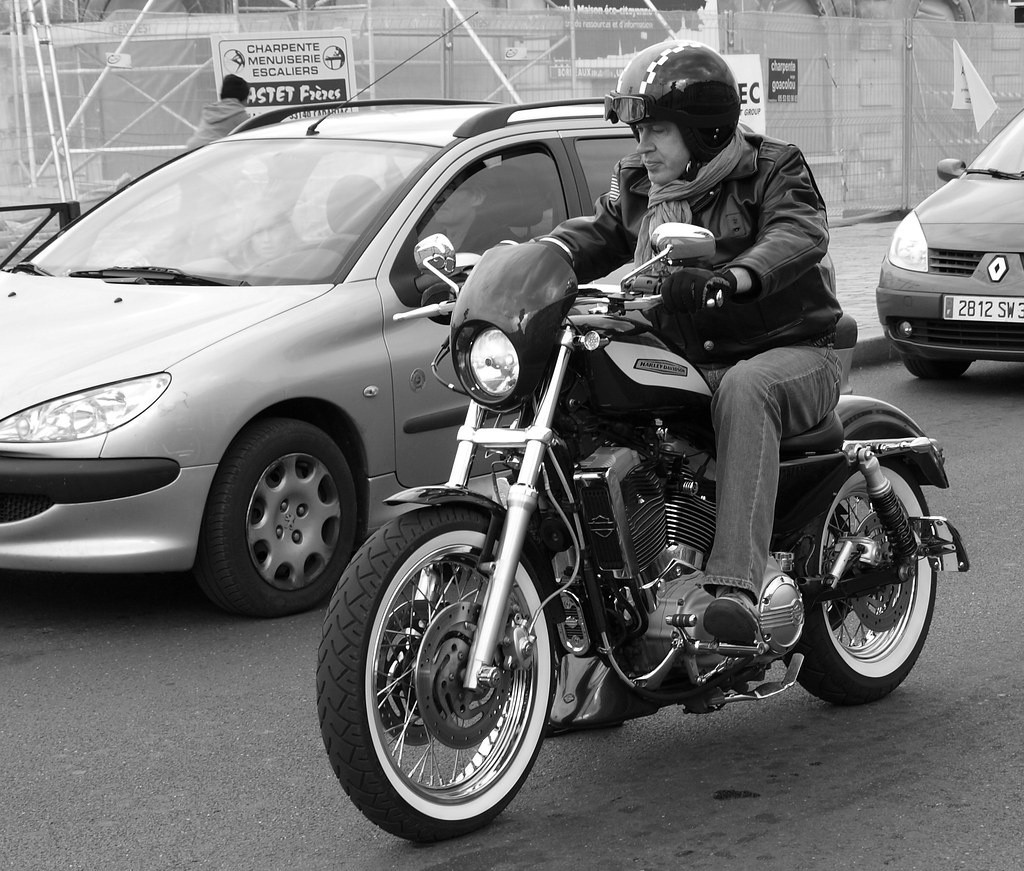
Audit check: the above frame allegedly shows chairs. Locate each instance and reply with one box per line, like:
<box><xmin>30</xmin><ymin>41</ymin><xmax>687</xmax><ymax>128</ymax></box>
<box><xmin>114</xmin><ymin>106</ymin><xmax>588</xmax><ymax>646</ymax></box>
<box><xmin>327</xmin><ymin>175</ymin><xmax>384</xmax><ymax>234</ymax></box>
<box><xmin>489</xmin><ymin>166</ymin><xmax>543</xmax><ymax>244</ymax></box>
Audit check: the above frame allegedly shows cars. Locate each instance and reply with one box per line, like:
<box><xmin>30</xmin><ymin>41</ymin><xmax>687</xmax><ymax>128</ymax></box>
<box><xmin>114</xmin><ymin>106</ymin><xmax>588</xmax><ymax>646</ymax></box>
<box><xmin>877</xmin><ymin>109</ymin><xmax>1024</xmax><ymax>380</ymax></box>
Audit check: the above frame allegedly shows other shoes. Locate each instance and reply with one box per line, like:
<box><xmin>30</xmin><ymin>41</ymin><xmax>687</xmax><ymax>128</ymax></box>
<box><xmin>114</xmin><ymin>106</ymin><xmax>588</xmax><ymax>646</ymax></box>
<box><xmin>704</xmin><ymin>587</ymin><xmax>760</xmax><ymax>657</ymax></box>
<box><xmin>547</xmin><ymin>718</ymin><xmax>624</xmax><ymax>736</ymax></box>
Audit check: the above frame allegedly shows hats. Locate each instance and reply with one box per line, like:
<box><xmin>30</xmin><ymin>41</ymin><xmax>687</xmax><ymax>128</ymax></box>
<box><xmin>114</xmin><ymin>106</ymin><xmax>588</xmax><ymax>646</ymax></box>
<box><xmin>221</xmin><ymin>74</ymin><xmax>249</xmax><ymax>101</ymax></box>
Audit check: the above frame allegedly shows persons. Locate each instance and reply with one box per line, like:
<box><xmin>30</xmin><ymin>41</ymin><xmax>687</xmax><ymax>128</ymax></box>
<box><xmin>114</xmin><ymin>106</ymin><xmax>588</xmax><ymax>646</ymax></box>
<box><xmin>186</xmin><ymin>73</ymin><xmax>256</xmax><ymax>156</ymax></box>
<box><xmin>492</xmin><ymin>37</ymin><xmax>844</xmax><ymax>649</ymax></box>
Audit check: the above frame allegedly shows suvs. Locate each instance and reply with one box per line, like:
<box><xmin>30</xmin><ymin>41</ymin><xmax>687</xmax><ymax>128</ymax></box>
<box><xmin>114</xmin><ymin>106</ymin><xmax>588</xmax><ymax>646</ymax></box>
<box><xmin>0</xmin><ymin>94</ymin><xmax>642</xmax><ymax>618</ymax></box>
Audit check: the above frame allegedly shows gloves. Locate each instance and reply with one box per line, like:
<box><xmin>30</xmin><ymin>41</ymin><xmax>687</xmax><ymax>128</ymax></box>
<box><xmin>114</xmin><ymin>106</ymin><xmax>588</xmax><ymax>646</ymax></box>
<box><xmin>421</xmin><ymin>274</ymin><xmax>468</xmax><ymax>325</ymax></box>
<box><xmin>660</xmin><ymin>266</ymin><xmax>738</xmax><ymax>315</ymax></box>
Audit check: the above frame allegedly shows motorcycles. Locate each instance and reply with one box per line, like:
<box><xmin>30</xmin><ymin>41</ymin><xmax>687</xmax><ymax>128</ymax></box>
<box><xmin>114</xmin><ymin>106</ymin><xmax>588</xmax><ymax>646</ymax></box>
<box><xmin>314</xmin><ymin>221</ymin><xmax>970</xmax><ymax>841</ymax></box>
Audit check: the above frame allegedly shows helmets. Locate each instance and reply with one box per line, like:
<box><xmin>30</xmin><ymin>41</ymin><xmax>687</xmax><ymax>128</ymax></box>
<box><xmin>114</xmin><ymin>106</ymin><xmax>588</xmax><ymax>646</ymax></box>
<box><xmin>615</xmin><ymin>40</ymin><xmax>740</xmax><ymax>160</ymax></box>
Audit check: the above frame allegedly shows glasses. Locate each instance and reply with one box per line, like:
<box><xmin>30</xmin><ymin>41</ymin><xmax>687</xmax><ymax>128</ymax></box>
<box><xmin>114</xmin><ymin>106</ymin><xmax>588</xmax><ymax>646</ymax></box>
<box><xmin>603</xmin><ymin>92</ymin><xmax>653</xmax><ymax>124</ymax></box>
